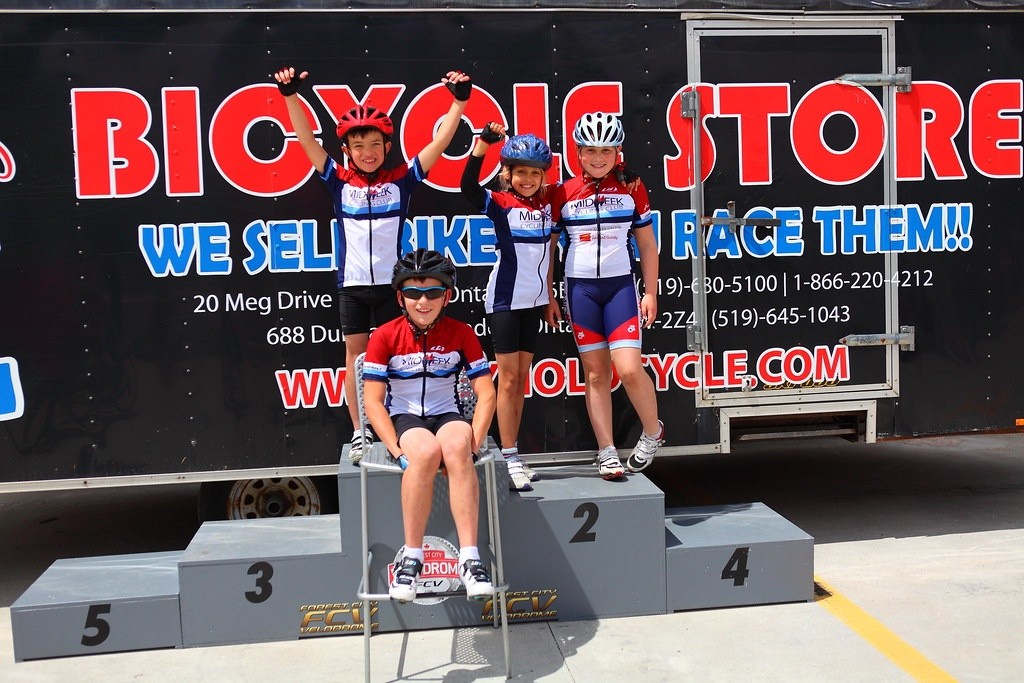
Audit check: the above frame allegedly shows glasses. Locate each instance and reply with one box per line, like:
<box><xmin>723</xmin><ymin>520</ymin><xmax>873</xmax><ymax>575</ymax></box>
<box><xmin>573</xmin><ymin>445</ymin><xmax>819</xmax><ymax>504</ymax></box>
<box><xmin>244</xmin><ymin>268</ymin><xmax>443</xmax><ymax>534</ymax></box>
<box><xmin>397</xmin><ymin>285</ymin><xmax>449</xmax><ymax>300</ymax></box>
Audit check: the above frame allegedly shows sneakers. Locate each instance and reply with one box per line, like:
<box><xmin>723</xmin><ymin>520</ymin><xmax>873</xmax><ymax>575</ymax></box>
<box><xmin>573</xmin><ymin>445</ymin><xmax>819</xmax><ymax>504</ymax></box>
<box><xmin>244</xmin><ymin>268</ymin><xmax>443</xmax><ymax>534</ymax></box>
<box><xmin>349</xmin><ymin>428</ymin><xmax>373</xmax><ymax>462</ymax></box>
<box><xmin>509</xmin><ymin>463</ymin><xmax>532</xmax><ymax>491</ymax></box>
<box><xmin>592</xmin><ymin>450</ymin><xmax>625</xmax><ymax>480</ymax></box>
<box><xmin>519</xmin><ymin>461</ymin><xmax>538</xmax><ymax>481</ymax></box>
<box><xmin>458</xmin><ymin>558</ymin><xmax>493</xmax><ymax>596</ymax></box>
<box><xmin>389</xmin><ymin>556</ymin><xmax>423</xmax><ymax>601</ymax></box>
<box><xmin>627</xmin><ymin>419</ymin><xmax>665</xmax><ymax>472</ymax></box>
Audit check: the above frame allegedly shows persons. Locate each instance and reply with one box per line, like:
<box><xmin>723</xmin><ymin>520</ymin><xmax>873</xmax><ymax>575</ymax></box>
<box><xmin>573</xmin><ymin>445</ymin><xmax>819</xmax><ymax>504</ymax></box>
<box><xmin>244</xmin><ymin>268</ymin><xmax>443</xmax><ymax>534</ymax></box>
<box><xmin>271</xmin><ymin>65</ymin><xmax>473</xmax><ymax>464</ymax></box>
<box><xmin>460</xmin><ymin>120</ymin><xmax>641</xmax><ymax>491</ymax></box>
<box><xmin>359</xmin><ymin>248</ymin><xmax>497</xmax><ymax>602</ymax></box>
<box><xmin>544</xmin><ymin>111</ymin><xmax>666</xmax><ymax>480</ymax></box>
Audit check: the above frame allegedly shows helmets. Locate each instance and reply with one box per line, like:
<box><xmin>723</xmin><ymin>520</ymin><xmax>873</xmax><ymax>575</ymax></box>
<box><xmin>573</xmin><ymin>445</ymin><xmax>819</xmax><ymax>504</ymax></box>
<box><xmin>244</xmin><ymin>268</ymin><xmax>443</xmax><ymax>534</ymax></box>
<box><xmin>500</xmin><ymin>133</ymin><xmax>552</xmax><ymax>169</ymax></box>
<box><xmin>391</xmin><ymin>248</ymin><xmax>454</xmax><ymax>289</ymax></box>
<box><xmin>336</xmin><ymin>105</ymin><xmax>394</xmax><ymax>140</ymax></box>
<box><xmin>572</xmin><ymin>111</ymin><xmax>626</xmax><ymax>146</ymax></box>
